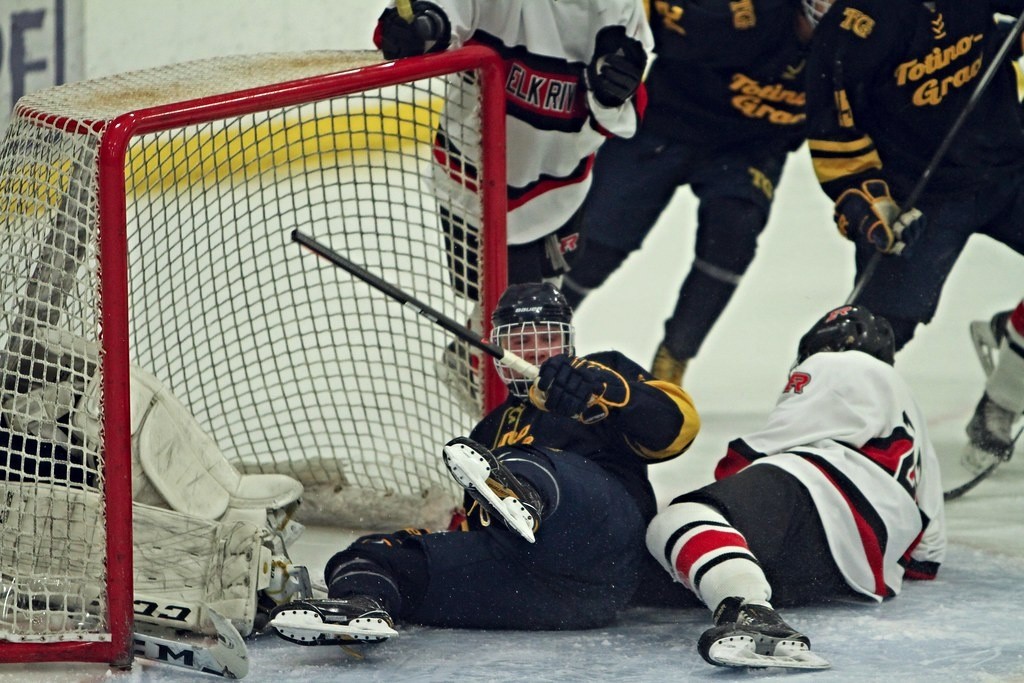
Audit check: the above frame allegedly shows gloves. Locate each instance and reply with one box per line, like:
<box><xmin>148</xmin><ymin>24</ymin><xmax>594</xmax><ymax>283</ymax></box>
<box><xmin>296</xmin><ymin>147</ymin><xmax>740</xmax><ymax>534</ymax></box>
<box><xmin>577</xmin><ymin>24</ymin><xmax>649</xmax><ymax>107</ymax></box>
<box><xmin>373</xmin><ymin>1</ymin><xmax>452</xmax><ymax>60</ymax></box>
<box><xmin>538</xmin><ymin>354</ymin><xmax>631</xmax><ymax>425</ymax></box>
<box><xmin>834</xmin><ymin>178</ymin><xmax>927</xmax><ymax>258</ymax></box>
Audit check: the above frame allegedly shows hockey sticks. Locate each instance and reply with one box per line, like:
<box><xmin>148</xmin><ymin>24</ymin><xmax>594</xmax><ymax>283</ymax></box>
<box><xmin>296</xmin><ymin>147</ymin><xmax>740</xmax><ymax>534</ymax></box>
<box><xmin>289</xmin><ymin>228</ymin><xmax>541</xmax><ymax>382</ymax></box>
<box><xmin>13</xmin><ymin>603</ymin><xmax>250</xmax><ymax>680</ymax></box>
<box><xmin>395</xmin><ymin>0</ymin><xmax>414</xmax><ymax>26</ymax></box>
<box><xmin>839</xmin><ymin>9</ymin><xmax>1024</xmax><ymax>312</ymax></box>
<box><xmin>940</xmin><ymin>425</ymin><xmax>1024</xmax><ymax>502</ymax></box>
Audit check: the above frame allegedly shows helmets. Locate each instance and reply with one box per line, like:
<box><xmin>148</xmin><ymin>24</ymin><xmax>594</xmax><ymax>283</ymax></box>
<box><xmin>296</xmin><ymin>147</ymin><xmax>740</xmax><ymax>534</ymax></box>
<box><xmin>788</xmin><ymin>304</ymin><xmax>896</xmax><ymax>381</ymax></box>
<box><xmin>490</xmin><ymin>281</ymin><xmax>576</xmax><ymax>403</ymax></box>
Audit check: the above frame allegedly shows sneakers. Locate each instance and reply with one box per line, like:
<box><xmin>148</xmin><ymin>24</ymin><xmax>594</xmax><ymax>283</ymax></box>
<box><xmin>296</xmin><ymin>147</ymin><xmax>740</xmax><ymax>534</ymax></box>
<box><xmin>268</xmin><ymin>595</ymin><xmax>399</xmax><ymax>646</ymax></box>
<box><xmin>961</xmin><ymin>390</ymin><xmax>1017</xmax><ymax>480</ymax></box>
<box><xmin>442</xmin><ymin>436</ymin><xmax>545</xmax><ymax>544</ymax></box>
<box><xmin>697</xmin><ymin>595</ymin><xmax>831</xmax><ymax>675</ymax></box>
<box><xmin>651</xmin><ymin>343</ymin><xmax>688</xmax><ymax>389</ymax></box>
<box><xmin>970</xmin><ymin>309</ymin><xmax>1014</xmax><ymax>380</ymax></box>
<box><xmin>435</xmin><ymin>340</ymin><xmax>473</xmax><ymax>423</ymax></box>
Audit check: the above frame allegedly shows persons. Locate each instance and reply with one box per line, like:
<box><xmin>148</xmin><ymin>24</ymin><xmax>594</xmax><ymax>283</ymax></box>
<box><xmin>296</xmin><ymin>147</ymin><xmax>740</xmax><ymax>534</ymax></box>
<box><xmin>372</xmin><ymin>0</ymin><xmax>657</xmax><ymax>381</ymax></box>
<box><xmin>642</xmin><ymin>304</ymin><xmax>948</xmax><ymax>674</ymax></box>
<box><xmin>253</xmin><ymin>272</ymin><xmax>705</xmax><ymax>645</ymax></box>
<box><xmin>0</xmin><ymin>329</ymin><xmax>315</xmax><ymax>638</ymax></box>
<box><xmin>498</xmin><ymin>0</ymin><xmax>852</xmax><ymax>386</ymax></box>
<box><xmin>798</xmin><ymin>0</ymin><xmax>1024</xmax><ymax>479</ymax></box>
